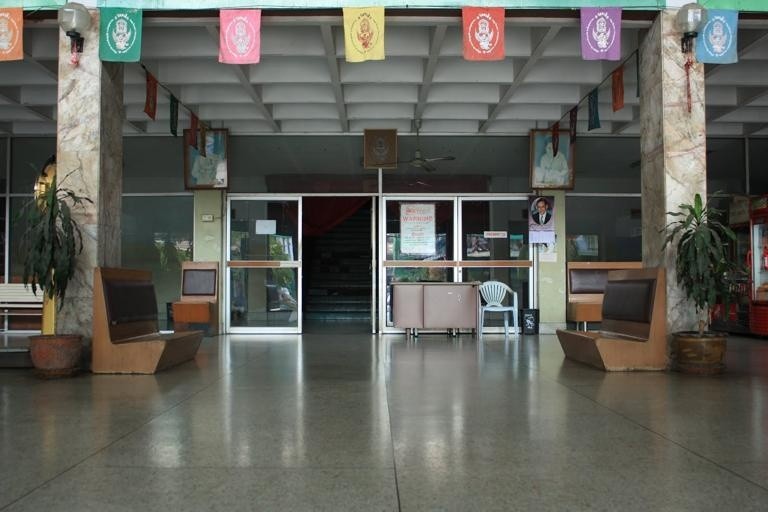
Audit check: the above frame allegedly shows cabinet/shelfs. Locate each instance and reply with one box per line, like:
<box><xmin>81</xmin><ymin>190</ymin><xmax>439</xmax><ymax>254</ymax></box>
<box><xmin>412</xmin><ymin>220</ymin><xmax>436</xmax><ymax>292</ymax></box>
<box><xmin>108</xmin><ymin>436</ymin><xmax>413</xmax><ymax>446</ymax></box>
<box><xmin>389</xmin><ymin>281</ymin><xmax>480</xmax><ymax>341</ymax></box>
<box><xmin>710</xmin><ymin>220</ymin><xmax>748</xmax><ymax>334</ymax></box>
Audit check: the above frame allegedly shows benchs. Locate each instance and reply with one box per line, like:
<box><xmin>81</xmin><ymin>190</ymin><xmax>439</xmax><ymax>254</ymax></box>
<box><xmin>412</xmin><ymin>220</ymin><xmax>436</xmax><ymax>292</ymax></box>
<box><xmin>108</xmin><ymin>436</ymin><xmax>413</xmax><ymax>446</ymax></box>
<box><xmin>91</xmin><ymin>266</ymin><xmax>204</xmax><ymax>375</ymax></box>
<box><xmin>564</xmin><ymin>261</ymin><xmax>639</xmax><ymax>330</ymax></box>
<box><xmin>0</xmin><ymin>283</ymin><xmax>45</xmax><ymax>332</ymax></box>
<box><xmin>555</xmin><ymin>265</ymin><xmax>668</xmax><ymax>370</ymax></box>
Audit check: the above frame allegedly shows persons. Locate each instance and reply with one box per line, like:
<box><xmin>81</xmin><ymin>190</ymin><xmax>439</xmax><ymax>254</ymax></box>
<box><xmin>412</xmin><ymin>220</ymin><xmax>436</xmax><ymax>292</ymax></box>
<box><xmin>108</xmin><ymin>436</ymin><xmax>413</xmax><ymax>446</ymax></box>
<box><xmin>535</xmin><ymin>137</ymin><xmax>568</xmax><ymax>185</ymax></box>
<box><xmin>192</xmin><ymin>135</ymin><xmax>223</xmax><ymax>185</ymax></box>
<box><xmin>532</xmin><ymin>198</ymin><xmax>552</xmax><ymax>225</ymax></box>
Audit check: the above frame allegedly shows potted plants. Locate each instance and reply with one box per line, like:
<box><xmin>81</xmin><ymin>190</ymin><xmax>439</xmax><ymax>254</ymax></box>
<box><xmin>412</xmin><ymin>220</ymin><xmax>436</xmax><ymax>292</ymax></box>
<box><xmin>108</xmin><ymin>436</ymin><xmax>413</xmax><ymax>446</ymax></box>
<box><xmin>14</xmin><ymin>163</ymin><xmax>94</xmax><ymax>380</ymax></box>
<box><xmin>658</xmin><ymin>190</ymin><xmax>748</xmax><ymax>377</ymax></box>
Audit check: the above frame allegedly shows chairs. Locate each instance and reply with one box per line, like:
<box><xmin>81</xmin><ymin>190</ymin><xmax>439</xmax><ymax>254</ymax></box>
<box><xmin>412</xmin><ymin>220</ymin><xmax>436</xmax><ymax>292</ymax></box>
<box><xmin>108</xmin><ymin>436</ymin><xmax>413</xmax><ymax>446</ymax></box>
<box><xmin>167</xmin><ymin>261</ymin><xmax>220</xmax><ymax>334</ymax></box>
<box><xmin>478</xmin><ymin>279</ymin><xmax>518</xmax><ymax>336</ymax></box>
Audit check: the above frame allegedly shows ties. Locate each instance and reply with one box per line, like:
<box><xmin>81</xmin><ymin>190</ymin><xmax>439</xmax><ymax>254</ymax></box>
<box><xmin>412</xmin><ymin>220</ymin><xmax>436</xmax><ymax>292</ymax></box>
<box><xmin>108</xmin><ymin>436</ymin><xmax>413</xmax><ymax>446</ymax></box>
<box><xmin>541</xmin><ymin>216</ymin><xmax>544</xmax><ymax>224</ymax></box>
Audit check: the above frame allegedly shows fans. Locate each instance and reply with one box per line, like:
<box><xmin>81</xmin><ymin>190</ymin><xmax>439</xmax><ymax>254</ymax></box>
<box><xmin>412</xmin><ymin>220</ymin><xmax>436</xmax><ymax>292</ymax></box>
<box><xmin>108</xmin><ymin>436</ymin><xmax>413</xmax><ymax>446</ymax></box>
<box><xmin>372</xmin><ymin>120</ymin><xmax>455</xmax><ymax>176</ymax></box>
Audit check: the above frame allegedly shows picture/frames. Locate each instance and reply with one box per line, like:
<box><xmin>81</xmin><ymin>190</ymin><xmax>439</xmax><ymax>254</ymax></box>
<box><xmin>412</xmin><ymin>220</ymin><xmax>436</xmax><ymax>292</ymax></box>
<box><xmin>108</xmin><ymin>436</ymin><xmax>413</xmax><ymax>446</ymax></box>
<box><xmin>529</xmin><ymin>128</ymin><xmax>574</xmax><ymax>192</ymax></box>
<box><xmin>363</xmin><ymin>128</ymin><xmax>397</xmax><ymax>171</ymax></box>
<box><xmin>181</xmin><ymin>128</ymin><xmax>228</xmax><ymax>190</ymax></box>
